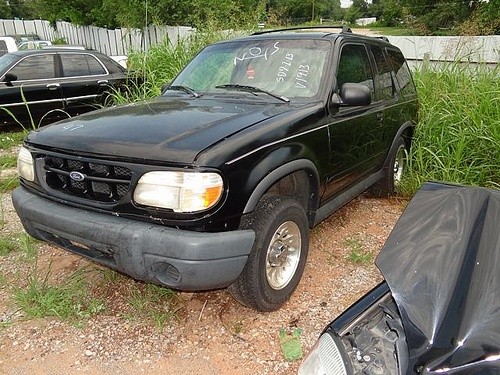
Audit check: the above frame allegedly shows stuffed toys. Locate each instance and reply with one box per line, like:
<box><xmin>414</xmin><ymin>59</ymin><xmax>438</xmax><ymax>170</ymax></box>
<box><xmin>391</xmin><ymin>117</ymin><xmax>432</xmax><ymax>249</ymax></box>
<box><xmin>247</xmin><ymin>64</ymin><xmax>255</xmax><ymax>82</ymax></box>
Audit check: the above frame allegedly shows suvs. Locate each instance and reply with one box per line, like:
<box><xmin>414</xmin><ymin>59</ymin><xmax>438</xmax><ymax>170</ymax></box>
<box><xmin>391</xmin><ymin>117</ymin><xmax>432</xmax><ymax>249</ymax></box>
<box><xmin>11</xmin><ymin>22</ymin><xmax>420</xmax><ymax>312</ymax></box>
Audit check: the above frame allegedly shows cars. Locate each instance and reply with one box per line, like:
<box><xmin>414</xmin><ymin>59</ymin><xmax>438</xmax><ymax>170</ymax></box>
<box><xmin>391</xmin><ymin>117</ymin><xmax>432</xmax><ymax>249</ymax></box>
<box><xmin>1</xmin><ymin>34</ymin><xmax>147</xmax><ymax>134</ymax></box>
